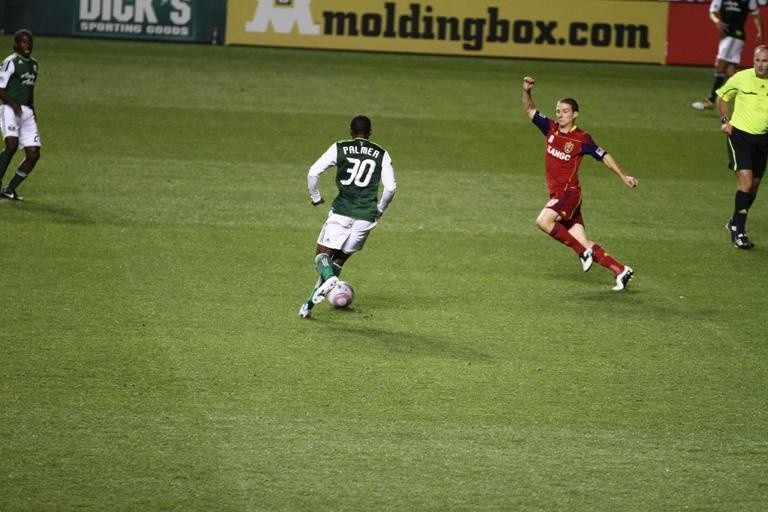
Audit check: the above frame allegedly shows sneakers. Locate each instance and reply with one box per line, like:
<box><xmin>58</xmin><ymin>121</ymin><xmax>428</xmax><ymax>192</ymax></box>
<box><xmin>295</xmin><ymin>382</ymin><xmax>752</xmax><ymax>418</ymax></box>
<box><xmin>725</xmin><ymin>217</ymin><xmax>738</xmax><ymax>242</ymax></box>
<box><xmin>692</xmin><ymin>98</ymin><xmax>715</xmax><ymax>110</ymax></box>
<box><xmin>612</xmin><ymin>265</ymin><xmax>634</xmax><ymax>291</ymax></box>
<box><xmin>299</xmin><ymin>304</ymin><xmax>311</xmax><ymax>319</ymax></box>
<box><xmin>580</xmin><ymin>248</ymin><xmax>594</xmax><ymax>272</ymax></box>
<box><xmin>312</xmin><ymin>276</ymin><xmax>339</xmax><ymax>305</ymax></box>
<box><xmin>2</xmin><ymin>188</ymin><xmax>24</xmax><ymax>201</ymax></box>
<box><xmin>734</xmin><ymin>234</ymin><xmax>754</xmax><ymax>249</ymax></box>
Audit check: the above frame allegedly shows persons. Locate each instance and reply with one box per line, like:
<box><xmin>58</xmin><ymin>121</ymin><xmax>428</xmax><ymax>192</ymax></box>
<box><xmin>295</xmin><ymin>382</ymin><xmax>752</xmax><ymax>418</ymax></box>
<box><xmin>519</xmin><ymin>75</ymin><xmax>638</xmax><ymax>292</ymax></box>
<box><xmin>690</xmin><ymin>0</ymin><xmax>763</xmax><ymax>110</ymax></box>
<box><xmin>298</xmin><ymin>114</ymin><xmax>397</xmax><ymax>319</ymax></box>
<box><xmin>714</xmin><ymin>43</ymin><xmax>767</xmax><ymax>250</ymax></box>
<box><xmin>0</xmin><ymin>27</ymin><xmax>41</xmax><ymax>202</ymax></box>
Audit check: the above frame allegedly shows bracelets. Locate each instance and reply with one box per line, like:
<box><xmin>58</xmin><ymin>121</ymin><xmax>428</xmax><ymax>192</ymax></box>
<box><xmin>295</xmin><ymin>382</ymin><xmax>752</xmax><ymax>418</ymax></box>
<box><xmin>720</xmin><ymin>115</ymin><xmax>730</xmax><ymax>122</ymax></box>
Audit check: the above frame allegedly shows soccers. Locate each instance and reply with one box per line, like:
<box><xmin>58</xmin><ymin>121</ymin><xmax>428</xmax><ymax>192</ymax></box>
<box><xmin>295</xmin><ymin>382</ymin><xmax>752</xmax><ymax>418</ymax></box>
<box><xmin>329</xmin><ymin>281</ymin><xmax>354</xmax><ymax>307</ymax></box>
